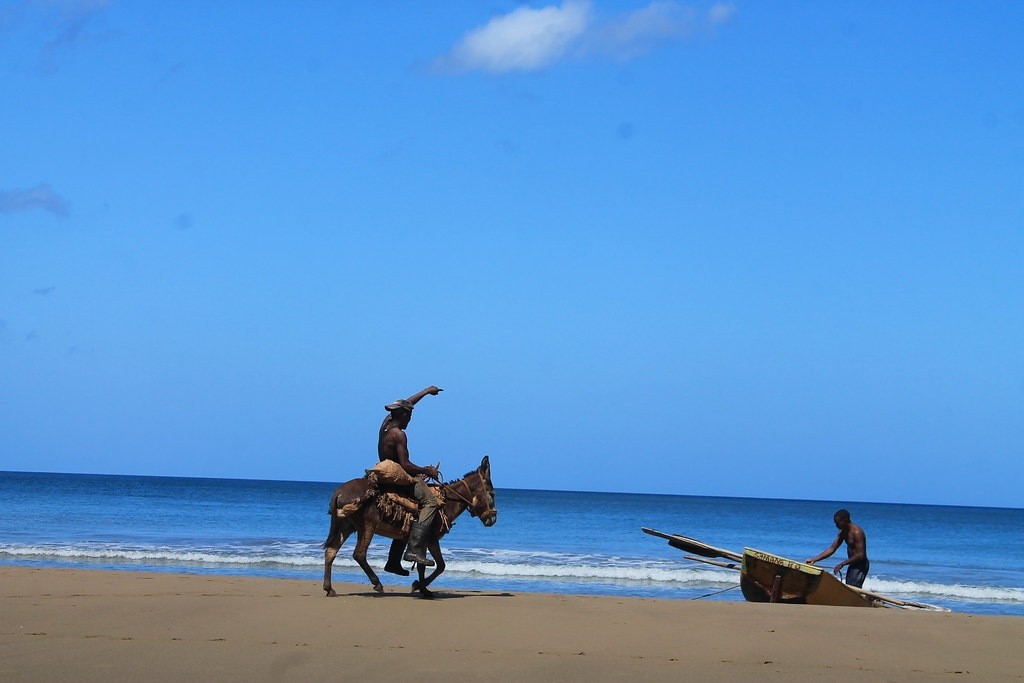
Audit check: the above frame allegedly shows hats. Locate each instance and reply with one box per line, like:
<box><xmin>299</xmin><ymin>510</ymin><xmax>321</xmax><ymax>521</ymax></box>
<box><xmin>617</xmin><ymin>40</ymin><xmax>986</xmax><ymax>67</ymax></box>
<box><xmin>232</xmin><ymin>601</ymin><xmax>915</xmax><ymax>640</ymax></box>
<box><xmin>385</xmin><ymin>399</ymin><xmax>414</xmax><ymax>413</ymax></box>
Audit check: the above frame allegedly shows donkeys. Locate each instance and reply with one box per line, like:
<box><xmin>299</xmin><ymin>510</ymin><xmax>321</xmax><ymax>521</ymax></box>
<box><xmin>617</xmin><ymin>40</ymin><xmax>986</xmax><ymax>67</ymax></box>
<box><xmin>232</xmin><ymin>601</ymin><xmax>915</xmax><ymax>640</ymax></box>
<box><xmin>322</xmin><ymin>455</ymin><xmax>498</xmax><ymax>599</ymax></box>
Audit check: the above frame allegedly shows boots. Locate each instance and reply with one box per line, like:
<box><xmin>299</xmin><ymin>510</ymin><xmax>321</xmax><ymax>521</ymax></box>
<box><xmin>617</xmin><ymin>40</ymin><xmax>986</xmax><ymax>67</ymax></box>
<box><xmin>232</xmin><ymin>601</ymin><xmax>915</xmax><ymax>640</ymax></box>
<box><xmin>384</xmin><ymin>545</ymin><xmax>409</xmax><ymax>576</ymax></box>
<box><xmin>403</xmin><ymin>522</ymin><xmax>435</xmax><ymax>566</ymax></box>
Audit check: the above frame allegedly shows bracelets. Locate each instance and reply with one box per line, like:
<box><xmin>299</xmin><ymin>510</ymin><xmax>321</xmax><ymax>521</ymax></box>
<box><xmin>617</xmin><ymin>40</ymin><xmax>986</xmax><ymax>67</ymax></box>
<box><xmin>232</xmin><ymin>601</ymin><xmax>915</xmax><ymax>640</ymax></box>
<box><xmin>841</xmin><ymin>562</ymin><xmax>845</xmax><ymax>567</ymax></box>
<box><xmin>424</xmin><ymin>388</ymin><xmax>430</xmax><ymax>394</ymax></box>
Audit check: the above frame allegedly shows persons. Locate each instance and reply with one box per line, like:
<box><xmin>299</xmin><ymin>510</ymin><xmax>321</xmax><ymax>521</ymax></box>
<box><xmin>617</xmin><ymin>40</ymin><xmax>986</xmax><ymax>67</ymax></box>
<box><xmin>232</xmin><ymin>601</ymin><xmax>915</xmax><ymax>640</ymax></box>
<box><xmin>804</xmin><ymin>509</ymin><xmax>870</xmax><ymax>589</ymax></box>
<box><xmin>377</xmin><ymin>385</ymin><xmax>445</xmax><ymax>576</ymax></box>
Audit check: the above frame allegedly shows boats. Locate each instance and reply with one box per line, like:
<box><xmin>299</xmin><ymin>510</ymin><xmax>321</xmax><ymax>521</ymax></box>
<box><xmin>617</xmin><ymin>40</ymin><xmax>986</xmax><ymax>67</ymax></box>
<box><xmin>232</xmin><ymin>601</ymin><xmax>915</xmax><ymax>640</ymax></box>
<box><xmin>741</xmin><ymin>546</ymin><xmax>873</xmax><ymax>607</ymax></box>
